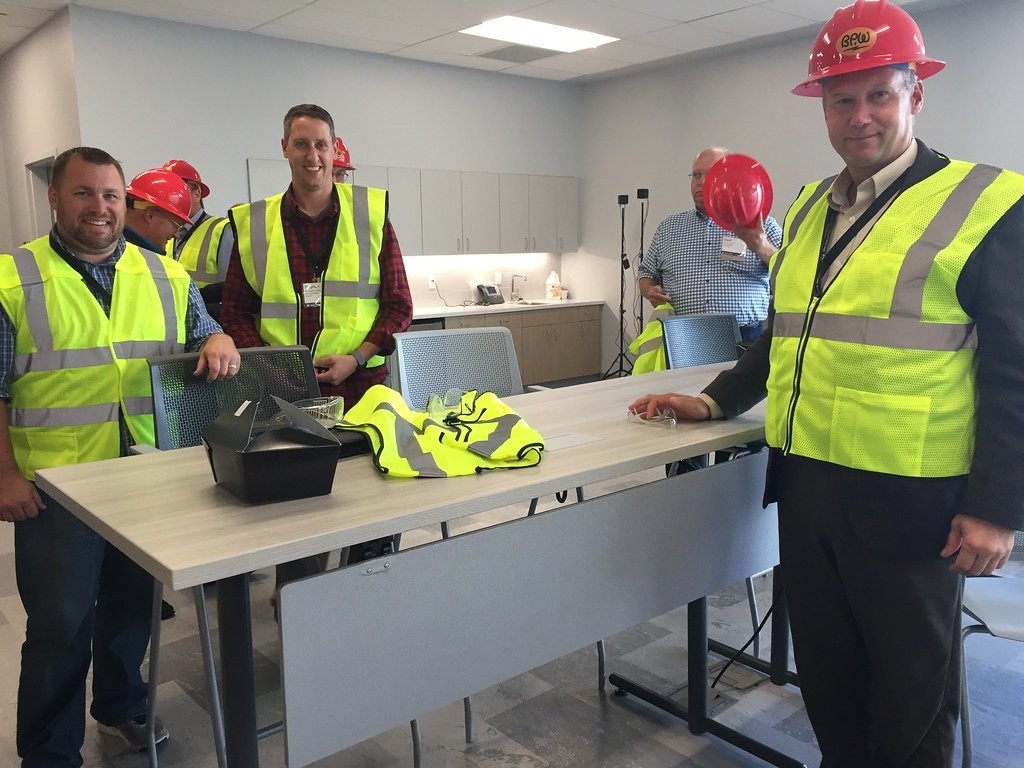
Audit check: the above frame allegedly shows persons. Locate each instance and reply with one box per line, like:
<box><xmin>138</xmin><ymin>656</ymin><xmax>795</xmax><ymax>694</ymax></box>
<box><xmin>119</xmin><ymin>166</ymin><xmax>195</xmax><ymax>257</ymax></box>
<box><xmin>628</xmin><ymin>0</ymin><xmax>1024</xmax><ymax>767</ymax></box>
<box><xmin>217</xmin><ymin>105</ymin><xmax>414</xmax><ymax>627</ymax></box>
<box><xmin>636</xmin><ymin>146</ymin><xmax>783</xmax><ymax>481</ymax></box>
<box><xmin>332</xmin><ymin>136</ymin><xmax>357</xmax><ymax>184</ymax></box>
<box><xmin>157</xmin><ymin>159</ymin><xmax>225</xmax><ymax>448</ymax></box>
<box><xmin>0</xmin><ymin>147</ymin><xmax>242</xmax><ymax>768</ymax></box>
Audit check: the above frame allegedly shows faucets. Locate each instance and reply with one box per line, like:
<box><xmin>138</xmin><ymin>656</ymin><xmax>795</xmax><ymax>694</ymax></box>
<box><xmin>510</xmin><ymin>274</ymin><xmax>527</xmax><ymax>302</ymax></box>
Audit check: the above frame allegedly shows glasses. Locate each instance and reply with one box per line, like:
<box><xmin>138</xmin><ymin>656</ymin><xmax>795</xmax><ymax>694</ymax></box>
<box><xmin>332</xmin><ymin>172</ymin><xmax>349</xmax><ymax>181</ymax></box>
<box><xmin>426</xmin><ymin>388</ymin><xmax>487</xmax><ymax>423</ymax></box>
<box><xmin>689</xmin><ymin>172</ymin><xmax>707</xmax><ymax>181</ymax></box>
<box><xmin>154</xmin><ymin>205</ymin><xmax>185</xmax><ymax>234</ymax></box>
<box><xmin>627</xmin><ymin>406</ymin><xmax>677</xmax><ymax>429</ymax></box>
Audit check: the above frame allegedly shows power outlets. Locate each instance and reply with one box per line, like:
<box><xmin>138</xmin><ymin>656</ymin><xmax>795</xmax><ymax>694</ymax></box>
<box><xmin>428</xmin><ymin>277</ymin><xmax>438</xmax><ymax>289</ymax></box>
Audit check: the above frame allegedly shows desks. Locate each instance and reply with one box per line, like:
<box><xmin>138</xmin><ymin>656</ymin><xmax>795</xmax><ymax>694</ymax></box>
<box><xmin>34</xmin><ymin>358</ymin><xmax>820</xmax><ymax>768</ymax></box>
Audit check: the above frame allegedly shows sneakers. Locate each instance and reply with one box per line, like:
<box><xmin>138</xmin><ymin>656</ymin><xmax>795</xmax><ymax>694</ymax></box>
<box><xmin>97</xmin><ymin>712</ymin><xmax>170</xmax><ymax>753</ymax></box>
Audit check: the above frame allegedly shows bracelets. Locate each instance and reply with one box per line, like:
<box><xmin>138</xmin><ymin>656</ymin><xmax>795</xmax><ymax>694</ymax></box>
<box><xmin>348</xmin><ymin>349</ymin><xmax>366</xmax><ymax>367</ymax></box>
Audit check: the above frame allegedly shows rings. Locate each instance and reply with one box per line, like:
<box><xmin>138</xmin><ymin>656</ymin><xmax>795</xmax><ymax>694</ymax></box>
<box><xmin>229</xmin><ymin>365</ymin><xmax>236</xmax><ymax>368</ymax></box>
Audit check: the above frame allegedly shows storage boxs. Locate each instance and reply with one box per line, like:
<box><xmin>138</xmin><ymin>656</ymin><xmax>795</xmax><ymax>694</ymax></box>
<box><xmin>197</xmin><ymin>394</ymin><xmax>341</xmax><ymax>505</ymax></box>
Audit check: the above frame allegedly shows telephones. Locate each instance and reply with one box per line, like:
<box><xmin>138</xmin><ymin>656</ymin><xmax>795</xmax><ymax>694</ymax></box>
<box><xmin>477</xmin><ymin>285</ymin><xmax>505</xmax><ymax>304</ymax></box>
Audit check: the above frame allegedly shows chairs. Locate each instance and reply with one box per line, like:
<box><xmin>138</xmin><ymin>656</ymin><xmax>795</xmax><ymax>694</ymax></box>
<box><xmin>659</xmin><ymin>315</ymin><xmax>746</xmax><ymax>370</ymax></box>
<box><xmin>393</xmin><ymin>327</ymin><xmax>607</xmax><ymax>744</ymax></box>
<box><xmin>146</xmin><ymin>345</ymin><xmax>425</xmax><ymax>768</ymax></box>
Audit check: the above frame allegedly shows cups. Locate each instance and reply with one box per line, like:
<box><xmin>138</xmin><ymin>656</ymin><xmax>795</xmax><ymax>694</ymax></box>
<box><xmin>561</xmin><ymin>291</ymin><xmax>568</xmax><ymax>300</ymax></box>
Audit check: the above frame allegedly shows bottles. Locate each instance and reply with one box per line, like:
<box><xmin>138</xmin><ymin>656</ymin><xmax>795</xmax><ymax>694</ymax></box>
<box><xmin>545</xmin><ymin>271</ymin><xmax>560</xmax><ymax>300</ymax></box>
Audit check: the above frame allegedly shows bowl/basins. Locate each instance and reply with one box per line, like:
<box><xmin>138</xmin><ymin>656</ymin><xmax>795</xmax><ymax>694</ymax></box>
<box><xmin>289</xmin><ymin>395</ymin><xmax>344</xmax><ymax>427</ymax></box>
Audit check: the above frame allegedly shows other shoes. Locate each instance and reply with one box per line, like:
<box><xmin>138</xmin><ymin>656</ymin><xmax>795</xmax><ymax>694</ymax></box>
<box><xmin>161</xmin><ymin>599</ymin><xmax>175</xmax><ymax>620</ymax></box>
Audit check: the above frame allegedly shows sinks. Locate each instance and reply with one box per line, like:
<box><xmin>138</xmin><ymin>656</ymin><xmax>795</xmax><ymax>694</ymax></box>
<box><xmin>507</xmin><ymin>302</ymin><xmax>551</xmax><ymax>306</ymax></box>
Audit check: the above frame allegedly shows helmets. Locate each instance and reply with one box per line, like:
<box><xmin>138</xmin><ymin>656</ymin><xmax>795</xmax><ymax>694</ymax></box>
<box><xmin>333</xmin><ymin>137</ymin><xmax>357</xmax><ymax>170</ymax></box>
<box><xmin>702</xmin><ymin>154</ymin><xmax>773</xmax><ymax>231</ymax></box>
<box><xmin>791</xmin><ymin>0</ymin><xmax>947</xmax><ymax>97</ymax></box>
<box><xmin>125</xmin><ymin>168</ymin><xmax>195</xmax><ymax>226</ymax></box>
<box><xmin>162</xmin><ymin>160</ymin><xmax>210</xmax><ymax>198</ymax></box>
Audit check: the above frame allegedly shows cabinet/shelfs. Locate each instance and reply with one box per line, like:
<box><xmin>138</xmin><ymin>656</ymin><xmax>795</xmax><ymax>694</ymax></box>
<box><xmin>412</xmin><ymin>304</ymin><xmax>604</xmax><ymax>390</ymax></box>
<box><xmin>346</xmin><ymin>165</ymin><xmax>579</xmax><ymax>254</ymax></box>
